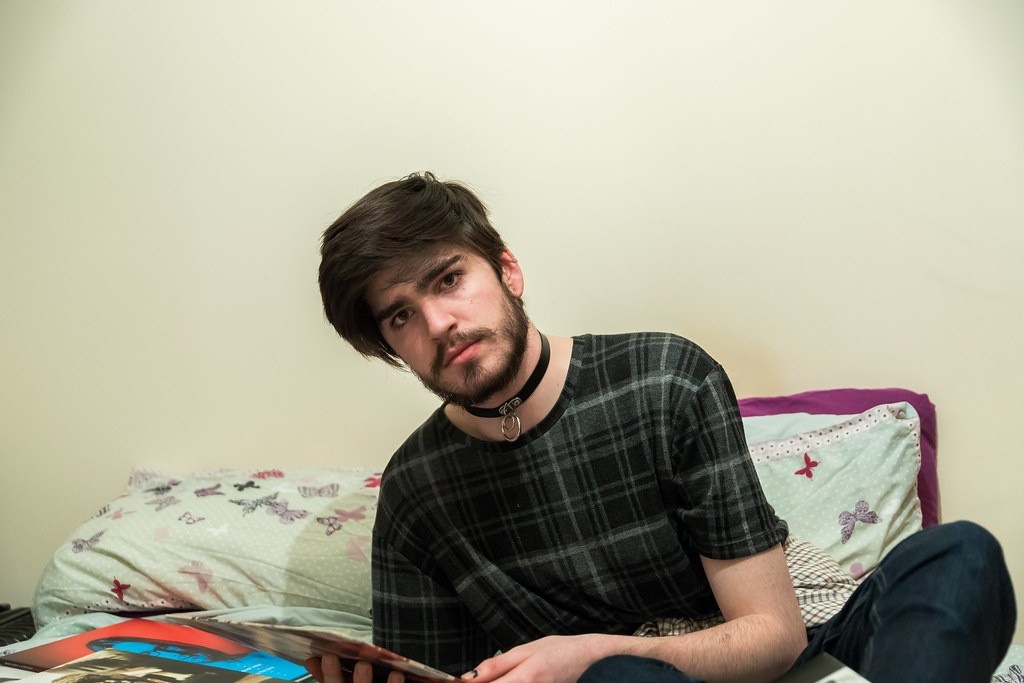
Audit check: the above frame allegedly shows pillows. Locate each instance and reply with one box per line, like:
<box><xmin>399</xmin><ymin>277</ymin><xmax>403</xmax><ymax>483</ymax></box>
<box><xmin>737</xmin><ymin>388</ymin><xmax>942</xmax><ymax>529</ymax></box>
<box><xmin>748</xmin><ymin>401</ymin><xmax>924</xmax><ymax>584</ymax></box>
<box><xmin>32</xmin><ymin>464</ymin><xmax>383</xmax><ymax>633</ymax></box>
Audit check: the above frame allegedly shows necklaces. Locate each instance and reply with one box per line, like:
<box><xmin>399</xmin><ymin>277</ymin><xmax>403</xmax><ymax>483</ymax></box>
<box><xmin>460</xmin><ymin>328</ymin><xmax>553</xmax><ymax>443</ymax></box>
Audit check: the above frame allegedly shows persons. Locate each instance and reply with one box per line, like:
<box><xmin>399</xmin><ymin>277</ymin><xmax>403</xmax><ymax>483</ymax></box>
<box><xmin>86</xmin><ymin>636</ymin><xmax>308</xmax><ymax>681</ymax></box>
<box><xmin>318</xmin><ymin>171</ymin><xmax>1017</xmax><ymax>683</ymax></box>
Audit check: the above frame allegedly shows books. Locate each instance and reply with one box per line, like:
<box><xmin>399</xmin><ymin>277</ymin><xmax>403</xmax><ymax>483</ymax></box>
<box><xmin>0</xmin><ymin>614</ymin><xmax>463</xmax><ymax>683</ymax></box>
